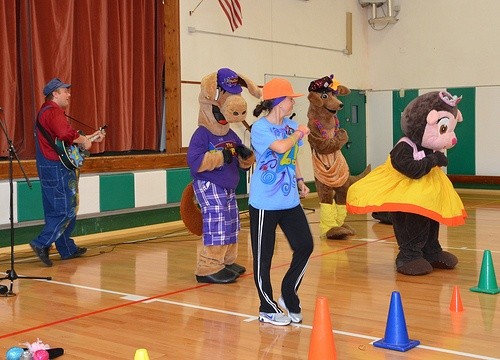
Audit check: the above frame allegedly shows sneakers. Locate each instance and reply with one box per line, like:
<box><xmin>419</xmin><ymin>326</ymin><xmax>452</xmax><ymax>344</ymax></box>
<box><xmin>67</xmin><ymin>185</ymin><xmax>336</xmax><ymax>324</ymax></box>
<box><xmin>259</xmin><ymin>311</ymin><xmax>292</xmax><ymax>326</ymax></box>
<box><xmin>279</xmin><ymin>297</ymin><xmax>302</xmax><ymax>323</ymax></box>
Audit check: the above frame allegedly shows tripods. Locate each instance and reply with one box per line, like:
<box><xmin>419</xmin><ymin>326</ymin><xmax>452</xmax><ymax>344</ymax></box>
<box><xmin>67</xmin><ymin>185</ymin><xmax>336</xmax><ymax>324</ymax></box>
<box><xmin>0</xmin><ymin>118</ymin><xmax>52</xmax><ymax>295</ymax></box>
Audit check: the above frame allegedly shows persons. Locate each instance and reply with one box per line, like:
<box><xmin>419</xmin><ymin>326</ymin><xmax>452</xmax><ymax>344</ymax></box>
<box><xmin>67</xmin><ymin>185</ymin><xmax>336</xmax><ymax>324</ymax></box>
<box><xmin>29</xmin><ymin>78</ymin><xmax>106</xmax><ymax>266</ymax></box>
<box><xmin>248</xmin><ymin>78</ymin><xmax>314</xmax><ymax>326</ymax></box>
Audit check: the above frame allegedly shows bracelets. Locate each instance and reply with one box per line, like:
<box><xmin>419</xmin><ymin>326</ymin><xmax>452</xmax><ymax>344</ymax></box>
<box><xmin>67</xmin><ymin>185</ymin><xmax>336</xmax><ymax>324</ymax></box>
<box><xmin>297</xmin><ymin>178</ymin><xmax>303</xmax><ymax>182</ymax></box>
<box><xmin>290</xmin><ymin>135</ymin><xmax>297</xmax><ymax>144</ymax></box>
<box><xmin>296</xmin><ymin>130</ymin><xmax>304</xmax><ymax>140</ymax></box>
<box><xmin>82</xmin><ymin>136</ymin><xmax>86</xmax><ymax>144</ymax></box>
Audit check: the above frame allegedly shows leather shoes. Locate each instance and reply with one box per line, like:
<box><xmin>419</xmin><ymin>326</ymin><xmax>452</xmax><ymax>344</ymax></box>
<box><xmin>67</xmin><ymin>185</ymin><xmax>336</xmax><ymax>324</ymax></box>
<box><xmin>30</xmin><ymin>243</ymin><xmax>53</xmax><ymax>266</ymax></box>
<box><xmin>71</xmin><ymin>248</ymin><xmax>87</xmax><ymax>257</ymax></box>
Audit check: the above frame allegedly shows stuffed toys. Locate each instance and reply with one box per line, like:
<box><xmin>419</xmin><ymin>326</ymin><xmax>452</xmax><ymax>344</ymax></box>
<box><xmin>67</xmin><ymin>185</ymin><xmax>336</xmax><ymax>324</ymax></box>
<box><xmin>346</xmin><ymin>91</ymin><xmax>467</xmax><ymax>276</ymax></box>
<box><xmin>180</xmin><ymin>68</ymin><xmax>262</xmax><ymax>283</ymax></box>
<box><xmin>307</xmin><ymin>75</ymin><xmax>372</xmax><ymax>239</ymax></box>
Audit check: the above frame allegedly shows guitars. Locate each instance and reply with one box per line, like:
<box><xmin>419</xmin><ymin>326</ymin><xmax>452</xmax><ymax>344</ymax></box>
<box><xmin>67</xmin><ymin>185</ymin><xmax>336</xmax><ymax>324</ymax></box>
<box><xmin>54</xmin><ymin>123</ymin><xmax>109</xmax><ymax>172</ymax></box>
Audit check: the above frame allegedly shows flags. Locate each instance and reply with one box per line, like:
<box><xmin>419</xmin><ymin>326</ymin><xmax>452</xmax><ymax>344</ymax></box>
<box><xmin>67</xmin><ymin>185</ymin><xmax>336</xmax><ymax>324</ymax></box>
<box><xmin>217</xmin><ymin>0</ymin><xmax>243</xmax><ymax>32</ymax></box>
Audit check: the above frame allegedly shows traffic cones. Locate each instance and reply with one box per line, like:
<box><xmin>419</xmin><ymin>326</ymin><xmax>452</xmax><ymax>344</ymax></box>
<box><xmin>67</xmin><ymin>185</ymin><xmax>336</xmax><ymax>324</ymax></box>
<box><xmin>308</xmin><ymin>297</ymin><xmax>338</xmax><ymax>360</ymax></box>
<box><xmin>477</xmin><ymin>292</ymin><xmax>497</xmax><ymax>334</ymax></box>
<box><xmin>373</xmin><ymin>291</ymin><xmax>420</xmax><ymax>352</ymax></box>
<box><xmin>469</xmin><ymin>250</ymin><xmax>500</xmax><ymax>295</ymax></box>
<box><xmin>450</xmin><ymin>311</ymin><xmax>464</xmax><ymax>334</ymax></box>
<box><xmin>449</xmin><ymin>285</ymin><xmax>463</xmax><ymax>311</ymax></box>
<box><xmin>134</xmin><ymin>348</ymin><xmax>148</xmax><ymax>360</ymax></box>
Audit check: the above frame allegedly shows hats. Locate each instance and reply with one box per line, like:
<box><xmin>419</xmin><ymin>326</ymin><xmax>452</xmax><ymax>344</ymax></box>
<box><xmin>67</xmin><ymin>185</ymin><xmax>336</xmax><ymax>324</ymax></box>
<box><xmin>43</xmin><ymin>78</ymin><xmax>72</xmax><ymax>95</ymax></box>
<box><xmin>263</xmin><ymin>78</ymin><xmax>305</xmax><ymax>100</ymax></box>
